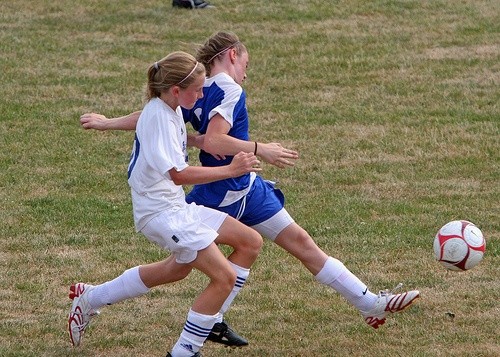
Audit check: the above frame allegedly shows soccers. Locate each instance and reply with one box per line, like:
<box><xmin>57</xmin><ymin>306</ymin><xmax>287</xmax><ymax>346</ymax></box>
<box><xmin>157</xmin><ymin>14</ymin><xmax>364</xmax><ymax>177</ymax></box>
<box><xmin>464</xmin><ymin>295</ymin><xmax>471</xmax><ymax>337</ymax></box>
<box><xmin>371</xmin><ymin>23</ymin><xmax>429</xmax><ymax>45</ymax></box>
<box><xmin>432</xmin><ymin>220</ymin><xmax>486</xmax><ymax>273</ymax></box>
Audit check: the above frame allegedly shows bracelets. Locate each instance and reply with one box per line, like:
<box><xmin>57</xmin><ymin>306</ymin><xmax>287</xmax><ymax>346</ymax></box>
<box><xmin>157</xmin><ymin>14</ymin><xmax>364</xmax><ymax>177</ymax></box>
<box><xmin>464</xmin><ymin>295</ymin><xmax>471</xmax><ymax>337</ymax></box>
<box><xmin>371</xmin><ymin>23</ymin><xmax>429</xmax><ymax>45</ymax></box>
<box><xmin>254</xmin><ymin>142</ymin><xmax>258</xmax><ymax>155</ymax></box>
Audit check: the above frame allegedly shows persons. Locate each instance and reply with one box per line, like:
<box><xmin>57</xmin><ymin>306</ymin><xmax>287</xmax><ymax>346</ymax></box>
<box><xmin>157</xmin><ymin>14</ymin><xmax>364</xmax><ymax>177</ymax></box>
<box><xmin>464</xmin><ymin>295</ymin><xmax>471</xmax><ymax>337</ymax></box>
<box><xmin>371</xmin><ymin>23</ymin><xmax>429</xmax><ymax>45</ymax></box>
<box><xmin>67</xmin><ymin>30</ymin><xmax>422</xmax><ymax>350</ymax></box>
<box><xmin>125</xmin><ymin>51</ymin><xmax>264</xmax><ymax>357</ymax></box>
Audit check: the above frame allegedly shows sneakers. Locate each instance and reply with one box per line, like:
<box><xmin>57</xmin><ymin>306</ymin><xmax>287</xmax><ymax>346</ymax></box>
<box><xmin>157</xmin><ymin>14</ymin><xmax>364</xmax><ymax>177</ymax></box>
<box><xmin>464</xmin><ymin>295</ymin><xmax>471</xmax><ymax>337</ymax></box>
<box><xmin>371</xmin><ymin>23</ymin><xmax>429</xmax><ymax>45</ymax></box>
<box><xmin>68</xmin><ymin>282</ymin><xmax>97</xmax><ymax>347</ymax></box>
<box><xmin>203</xmin><ymin>321</ymin><xmax>250</xmax><ymax>349</ymax></box>
<box><xmin>358</xmin><ymin>283</ymin><xmax>420</xmax><ymax>329</ymax></box>
